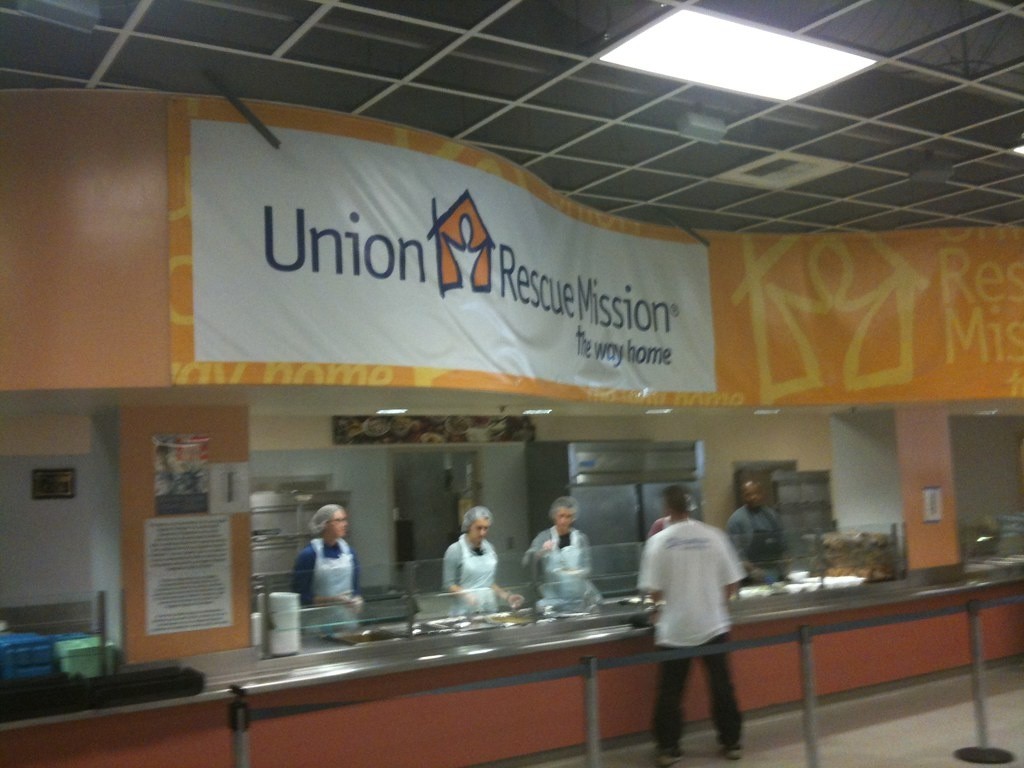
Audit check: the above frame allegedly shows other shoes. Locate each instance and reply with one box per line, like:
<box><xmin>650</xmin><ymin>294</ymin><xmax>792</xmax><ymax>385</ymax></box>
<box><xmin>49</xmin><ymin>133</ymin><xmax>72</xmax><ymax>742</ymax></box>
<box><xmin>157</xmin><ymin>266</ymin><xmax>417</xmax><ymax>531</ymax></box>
<box><xmin>657</xmin><ymin>744</ymin><xmax>685</xmax><ymax>765</ymax></box>
<box><xmin>720</xmin><ymin>744</ymin><xmax>742</xmax><ymax>757</ymax></box>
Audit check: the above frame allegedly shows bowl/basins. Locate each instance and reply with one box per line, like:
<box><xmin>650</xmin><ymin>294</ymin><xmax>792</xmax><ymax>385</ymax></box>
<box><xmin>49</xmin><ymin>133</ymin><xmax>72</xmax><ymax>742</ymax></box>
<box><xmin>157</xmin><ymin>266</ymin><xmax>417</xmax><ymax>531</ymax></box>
<box><xmin>783</xmin><ymin>584</ymin><xmax>804</xmax><ymax>594</ymax></box>
<box><xmin>268</xmin><ymin>612</ymin><xmax>300</xmax><ymax>630</ymax></box>
<box><xmin>268</xmin><ymin>592</ymin><xmax>300</xmax><ymax>613</ymax></box>
<box><xmin>788</xmin><ymin>571</ymin><xmax>808</xmax><ymax>582</ymax></box>
<box><xmin>757</xmin><ymin>590</ymin><xmax>773</xmax><ymax>597</ymax></box>
<box><xmin>738</xmin><ymin>589</ymin><xmax>752</xmax><ymax>600</ymax></box>
<box><xmin>266</xmin><ymin>630</ymin><xmax>301</xmax><ymax>657</ymax></box>
<box><xmin>802</xmin><ymin>582</ymin><xmax>822</xmax><ymax>592</ymax></box>
<box><xmin>801</xmin><ymin>577</ymin><xmax>821</xmax><ymax>583</ymax></box>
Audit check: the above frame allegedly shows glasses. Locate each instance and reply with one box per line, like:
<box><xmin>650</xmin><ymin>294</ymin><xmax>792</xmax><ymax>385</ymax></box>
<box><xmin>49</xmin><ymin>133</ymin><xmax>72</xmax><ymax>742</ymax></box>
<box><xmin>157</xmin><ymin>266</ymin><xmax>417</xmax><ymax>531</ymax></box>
<box><xmin>327</xmin><ymin>517</ymin><xmax>347</xmax><ymax>524</ymax></box>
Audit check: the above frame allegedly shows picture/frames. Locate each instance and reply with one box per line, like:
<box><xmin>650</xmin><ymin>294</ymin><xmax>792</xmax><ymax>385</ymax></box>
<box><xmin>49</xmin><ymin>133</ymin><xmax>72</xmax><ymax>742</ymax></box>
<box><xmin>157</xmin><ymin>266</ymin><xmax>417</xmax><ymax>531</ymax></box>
<box><xmin>30</xmin><ymin>467</ymin><xmax>75</xmax><ymax>500</ymax></box>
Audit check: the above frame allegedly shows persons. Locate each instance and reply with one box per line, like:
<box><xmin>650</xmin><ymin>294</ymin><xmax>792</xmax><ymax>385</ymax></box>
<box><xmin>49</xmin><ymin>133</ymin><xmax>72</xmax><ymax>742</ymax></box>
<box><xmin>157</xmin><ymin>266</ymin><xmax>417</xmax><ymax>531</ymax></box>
<box><xmin>291</xmin><ymin>504</ymin><xmax>359</xmax><ymax>605</ymax></box>
<box><xmin>441</xmin><ymin>504</ymin><xmax>525</xmax><ymax>616</ymax></box>
<box><xmin>647</xmin><ymin>493</ymin><xmax>697</xmax><ymax>540</ymax></box>
<box><xmin>726</xmin><ymin>476</ymin><xmax>788</xmax><ymax>587</ymax></box>
<box><xmin>636</xmin><ymin>484</ymin><xmax>748</xmax><ymax>767</ymax></box>
<box><xmin>520</xmin><ymin>496</ymin><xmax>601</xmax><ymax>614</ymax></box>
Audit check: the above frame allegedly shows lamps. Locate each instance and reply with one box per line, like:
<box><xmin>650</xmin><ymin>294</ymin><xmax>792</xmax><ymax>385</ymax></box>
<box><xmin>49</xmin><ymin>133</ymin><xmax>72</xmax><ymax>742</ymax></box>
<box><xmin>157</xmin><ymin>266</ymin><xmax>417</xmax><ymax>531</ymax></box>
<box><xmin>676</xmin><ymin>112</ymin><xmax>728</xmax><ymax>147</ymax></box>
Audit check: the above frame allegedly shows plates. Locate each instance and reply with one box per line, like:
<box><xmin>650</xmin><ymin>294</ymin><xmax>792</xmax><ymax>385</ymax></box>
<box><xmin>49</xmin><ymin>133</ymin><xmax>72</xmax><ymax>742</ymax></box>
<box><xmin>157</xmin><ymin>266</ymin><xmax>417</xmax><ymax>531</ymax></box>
<box><xmin>553</xmin><ymin>568</ymin><xmax>584</xmax><ymax>576</ymax></box>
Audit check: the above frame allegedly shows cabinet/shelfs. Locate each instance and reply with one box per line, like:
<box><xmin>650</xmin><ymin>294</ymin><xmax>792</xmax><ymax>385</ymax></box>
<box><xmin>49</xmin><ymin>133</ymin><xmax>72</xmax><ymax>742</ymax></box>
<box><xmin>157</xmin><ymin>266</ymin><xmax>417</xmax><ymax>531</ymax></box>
<box><xmin>250</xmin><ymin>490</ymin><xmax>351</xmax><ymax>593</ymax></box>
